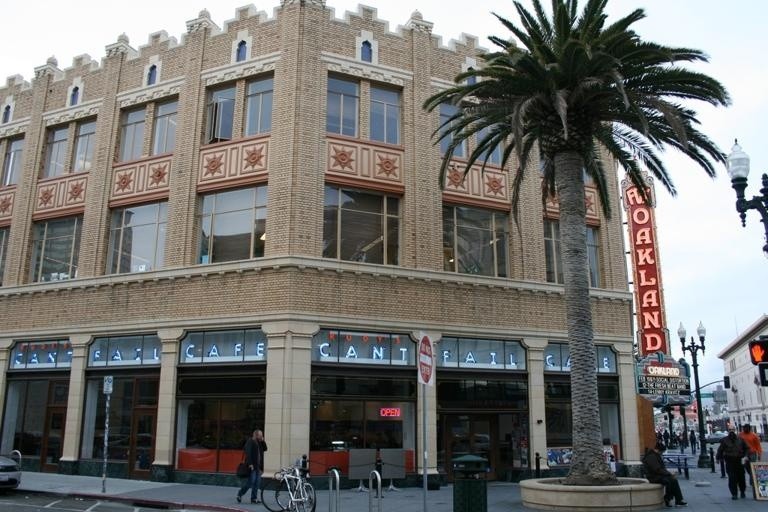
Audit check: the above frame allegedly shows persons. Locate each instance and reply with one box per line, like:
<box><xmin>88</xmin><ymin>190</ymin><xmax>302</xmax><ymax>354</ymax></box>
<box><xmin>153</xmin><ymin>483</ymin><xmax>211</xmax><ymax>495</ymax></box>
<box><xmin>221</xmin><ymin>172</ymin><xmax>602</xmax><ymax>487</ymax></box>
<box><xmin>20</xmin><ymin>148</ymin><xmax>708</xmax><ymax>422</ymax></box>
<box><xmin>641</xmin><ymin>442</ymin><xmax>688</xmax><ymax>507</ymax></box>
<box><xmin>656</xmin><ymin>429</ymin><xmax>689</xmax><ymax>449</ymax></box>
<box><xmin>715</xmin><ymin>439</ymin><xmax>730</xmax><ymax>478</ymax></box>
<box><xmin>715</xmin><ymin>421</ymin><xmax>751</xmax><ymax>500</ymax></box>
<box><xmin>690</xmin><ymin>430</ymin><xmax>697</xmax><ymax>453</ymax></box>
<box><xmin>737</xmin><ymin>424</ymin><xmax>763</xmax><ymax>486</ymax></box>
<box><xmin>235</xmin><ymin>429</ymin><xmax>268</xmax><ymax>505</ymax></box>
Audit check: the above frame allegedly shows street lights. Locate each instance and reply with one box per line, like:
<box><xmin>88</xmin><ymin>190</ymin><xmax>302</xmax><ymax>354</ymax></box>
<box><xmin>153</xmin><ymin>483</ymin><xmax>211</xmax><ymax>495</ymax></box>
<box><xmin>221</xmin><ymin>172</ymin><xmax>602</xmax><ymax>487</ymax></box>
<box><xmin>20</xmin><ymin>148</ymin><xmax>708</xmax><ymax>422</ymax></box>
<box><xmin>678</xmin><ymin>321</ymin><xmax>712</xmax><ymax>456</ymax></box>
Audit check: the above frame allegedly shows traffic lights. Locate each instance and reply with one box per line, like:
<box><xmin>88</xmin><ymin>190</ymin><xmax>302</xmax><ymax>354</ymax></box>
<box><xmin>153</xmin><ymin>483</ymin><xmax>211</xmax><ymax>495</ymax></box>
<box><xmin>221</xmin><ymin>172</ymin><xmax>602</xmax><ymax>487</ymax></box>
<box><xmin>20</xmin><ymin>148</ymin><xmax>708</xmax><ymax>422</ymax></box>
<box><xmin>749</xmin><ymin>339</ymin><xmax>767</xmax><ymax>366</ymax></box>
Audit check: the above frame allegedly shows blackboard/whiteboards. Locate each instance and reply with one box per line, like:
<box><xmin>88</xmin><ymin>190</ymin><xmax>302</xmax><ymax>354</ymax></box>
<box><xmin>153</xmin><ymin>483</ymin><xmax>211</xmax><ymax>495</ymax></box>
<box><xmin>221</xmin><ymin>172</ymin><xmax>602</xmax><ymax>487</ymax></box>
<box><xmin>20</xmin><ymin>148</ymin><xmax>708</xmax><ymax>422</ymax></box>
<box><xmin>750</xmin><ymin>462</ymin><xmax>768</xmax><ymax>500</ymax></box>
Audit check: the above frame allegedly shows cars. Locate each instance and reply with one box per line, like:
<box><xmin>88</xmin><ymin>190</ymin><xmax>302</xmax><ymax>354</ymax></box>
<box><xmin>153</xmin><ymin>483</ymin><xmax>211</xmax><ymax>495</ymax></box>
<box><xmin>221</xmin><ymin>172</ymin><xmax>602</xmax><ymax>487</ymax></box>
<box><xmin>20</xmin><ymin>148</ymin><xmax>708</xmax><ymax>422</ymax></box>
<box><xmin>107</xmin><ymin>433</ymin><xmax>153</xmax><ymax>457</ymax></box>
<box><xmin>0</xmin><ymin>455</ymin><xmax>21</xmax><ymax>491</ymax></box>
<box><xmin>15</xmin><ymin>431</ymin><xmax>61</xmax><ymax>455</ymax></box>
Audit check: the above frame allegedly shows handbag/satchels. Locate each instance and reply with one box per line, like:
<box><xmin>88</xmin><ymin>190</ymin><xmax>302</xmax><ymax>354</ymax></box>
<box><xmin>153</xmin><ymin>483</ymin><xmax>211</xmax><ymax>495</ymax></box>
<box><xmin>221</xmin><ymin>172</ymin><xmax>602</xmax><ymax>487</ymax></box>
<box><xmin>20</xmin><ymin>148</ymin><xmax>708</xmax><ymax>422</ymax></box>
<box><xmin>236</xmin><ymin>461</ymin><xmax>252</xmax><ymax>479</ymax></box>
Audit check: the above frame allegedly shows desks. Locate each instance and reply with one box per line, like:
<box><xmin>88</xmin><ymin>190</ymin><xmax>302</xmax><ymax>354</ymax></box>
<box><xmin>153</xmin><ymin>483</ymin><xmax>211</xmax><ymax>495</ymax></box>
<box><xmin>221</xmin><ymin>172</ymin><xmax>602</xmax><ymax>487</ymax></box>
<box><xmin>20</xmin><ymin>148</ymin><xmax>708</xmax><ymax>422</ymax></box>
<box><xmin>661</xmin><ymin>452</ymin><xmax>694</xmax><ymax>480</ymax></box>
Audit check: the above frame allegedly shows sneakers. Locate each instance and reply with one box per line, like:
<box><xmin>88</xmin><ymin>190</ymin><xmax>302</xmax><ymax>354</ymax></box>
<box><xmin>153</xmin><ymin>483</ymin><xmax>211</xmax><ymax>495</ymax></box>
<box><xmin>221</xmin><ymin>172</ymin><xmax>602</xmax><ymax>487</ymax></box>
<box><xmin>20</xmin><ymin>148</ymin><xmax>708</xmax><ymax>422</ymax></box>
<box><xmin>663</xmin><ymin>496</ymin><xmax>688</xmax><ymax>507</ymax></box>
<box><xmin>731</xmin><ymin>492</ymin><xmax>746</xmax><ymax>500</ymax></box>
<box><xmin>236</xmin><ymin>494</ymin><xmax>263</xmax><ymax>505</ymax></box>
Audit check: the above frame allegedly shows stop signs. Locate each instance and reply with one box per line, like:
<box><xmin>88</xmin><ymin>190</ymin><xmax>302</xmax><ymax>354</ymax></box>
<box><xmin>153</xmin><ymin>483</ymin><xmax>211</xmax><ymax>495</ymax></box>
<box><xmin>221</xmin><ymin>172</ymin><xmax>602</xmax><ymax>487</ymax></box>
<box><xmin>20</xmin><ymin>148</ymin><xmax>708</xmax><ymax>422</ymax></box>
<box><xmin>415</xmin><ymin>329</ymin><xmax>435</xmax><ymax>387</ymax></box>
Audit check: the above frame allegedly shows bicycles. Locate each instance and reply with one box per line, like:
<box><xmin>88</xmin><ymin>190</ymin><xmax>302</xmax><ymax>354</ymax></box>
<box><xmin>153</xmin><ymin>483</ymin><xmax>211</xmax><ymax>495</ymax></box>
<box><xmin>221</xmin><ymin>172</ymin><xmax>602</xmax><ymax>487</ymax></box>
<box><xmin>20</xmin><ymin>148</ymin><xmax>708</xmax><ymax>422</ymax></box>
<box><xmin>261</xmin><ymin>465</ymin><xmax>316</xmax><ymax>512</ymax></box>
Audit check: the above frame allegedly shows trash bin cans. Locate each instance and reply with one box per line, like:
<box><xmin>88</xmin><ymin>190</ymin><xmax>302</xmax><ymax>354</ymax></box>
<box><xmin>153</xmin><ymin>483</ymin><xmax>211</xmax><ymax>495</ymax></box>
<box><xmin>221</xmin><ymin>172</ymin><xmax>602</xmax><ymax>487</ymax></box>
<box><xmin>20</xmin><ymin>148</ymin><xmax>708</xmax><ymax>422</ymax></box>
<box><xmin>451</xmin><ymin>454</ymin><xmax>489</xmax><ymax>512</ymax></box>
